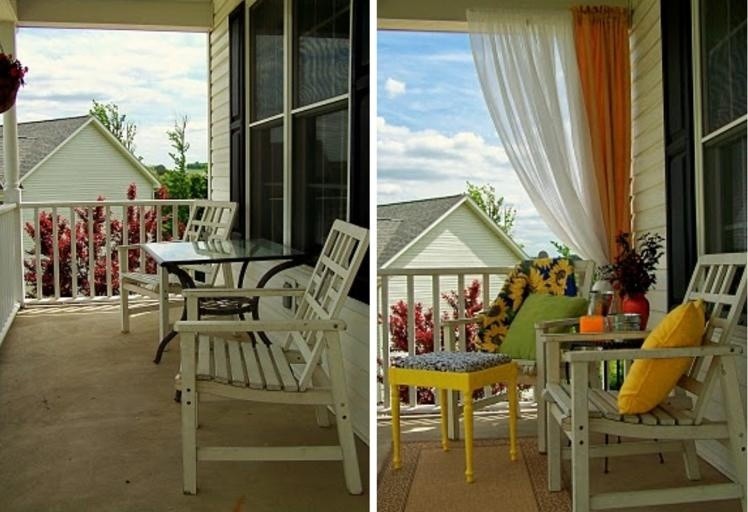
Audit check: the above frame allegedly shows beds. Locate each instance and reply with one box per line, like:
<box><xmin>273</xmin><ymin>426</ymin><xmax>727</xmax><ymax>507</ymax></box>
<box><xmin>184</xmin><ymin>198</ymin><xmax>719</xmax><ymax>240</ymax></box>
<box><xmin>138</xmin><ymin>238</ymin><xmax>307</xmax><ymax>365</ymax></box>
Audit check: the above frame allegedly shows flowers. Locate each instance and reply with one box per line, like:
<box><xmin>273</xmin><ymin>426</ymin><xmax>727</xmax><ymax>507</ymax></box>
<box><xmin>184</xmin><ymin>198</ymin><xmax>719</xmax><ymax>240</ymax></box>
<box><xmin>594</xmin><ymin>230</ymin><xmax>665</xmax><ymax>294</ymax></box>
<box><xmin>0</xmin><ymin>51</ymin><xmax>29</xmax><ymax>86</ymax></box>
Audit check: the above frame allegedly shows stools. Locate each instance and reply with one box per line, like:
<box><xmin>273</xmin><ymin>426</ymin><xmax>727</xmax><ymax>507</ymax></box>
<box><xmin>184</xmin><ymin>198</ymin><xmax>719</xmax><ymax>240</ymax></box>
<box><xmin>388</xmin><ymin>352</ymin><xmax>520</xmax><ymax>484</ymax></box>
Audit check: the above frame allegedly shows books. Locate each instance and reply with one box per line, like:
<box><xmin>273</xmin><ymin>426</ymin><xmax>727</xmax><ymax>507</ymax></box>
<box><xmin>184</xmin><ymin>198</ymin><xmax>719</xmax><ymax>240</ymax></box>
<box><xmin>608</xmin><ymin>312</ymin><xmax>641</xmax><ymax>331</ymax></box>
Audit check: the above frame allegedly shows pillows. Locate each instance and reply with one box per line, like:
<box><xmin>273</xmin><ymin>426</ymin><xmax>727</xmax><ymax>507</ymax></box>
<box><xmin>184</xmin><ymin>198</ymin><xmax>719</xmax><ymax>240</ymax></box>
<box><xmin>617</xmin><ymin>299</ymin><xmax>705</xmax><ymax>415</ymax></box>
<box><xmin>502</xmin><ymin>292</ymin><xmax>586</xmax><ymax>366</ymax></box>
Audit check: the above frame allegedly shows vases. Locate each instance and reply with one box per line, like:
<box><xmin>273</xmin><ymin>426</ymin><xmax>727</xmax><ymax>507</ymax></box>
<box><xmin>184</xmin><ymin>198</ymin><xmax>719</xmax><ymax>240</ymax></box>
<box><xmin>621</xmin><ymin>292</ymin><xmax>650</xmax><ymax>330</ymax></box>
<box><xmin>0</xmin><ymin>89</ymin><xmax>19</xmax><ymax>113</ymax></box>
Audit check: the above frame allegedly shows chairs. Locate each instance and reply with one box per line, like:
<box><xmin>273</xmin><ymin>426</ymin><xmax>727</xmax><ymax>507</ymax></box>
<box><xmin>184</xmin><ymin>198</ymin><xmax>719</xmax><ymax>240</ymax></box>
<box><xmin>442</xmin><ymin>252</ymin><xmax>596</xmax><ymax>453</ymax></box>
<box><xmin>173</xmin><ymin>218</ymin><xmax>370</xmax><ymax>497</ymax></box>
<box><xmin>541</xmin><ymin>247</ymin><xmax>748</xmax><ymax>511</ymax></box>
<box><xmin>116</xmin><ymin>199</ymin><xmax>239</xmax><ymax>353</ymax></box>
